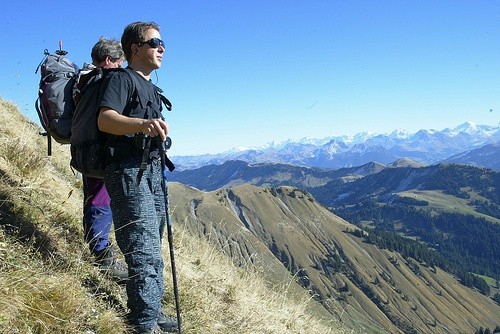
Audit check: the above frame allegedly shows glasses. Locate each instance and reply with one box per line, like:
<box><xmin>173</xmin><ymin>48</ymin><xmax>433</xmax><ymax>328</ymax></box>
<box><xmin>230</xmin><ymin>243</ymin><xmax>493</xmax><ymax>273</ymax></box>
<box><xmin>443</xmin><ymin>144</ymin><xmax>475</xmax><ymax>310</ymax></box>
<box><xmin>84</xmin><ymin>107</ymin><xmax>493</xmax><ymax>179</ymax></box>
<box><xmin>137</xmin><ymin>37</ymin><xmax>166</xmax><ymax>49</ymax></box>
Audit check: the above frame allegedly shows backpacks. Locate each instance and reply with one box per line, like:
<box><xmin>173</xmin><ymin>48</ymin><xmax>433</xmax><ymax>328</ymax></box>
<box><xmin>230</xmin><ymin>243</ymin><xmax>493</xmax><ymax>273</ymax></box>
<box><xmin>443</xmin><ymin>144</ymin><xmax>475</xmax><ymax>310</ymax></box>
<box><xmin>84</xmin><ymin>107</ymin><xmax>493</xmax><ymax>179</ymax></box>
<box><xmin>35</xmin><ymin>39</ymin><xmax>95</xmax><ymax>156</ymax></box>
<box><xmin>69</xmin><ymin>69</ymin><xmax>175</xmax><ymax>179</ymax></box>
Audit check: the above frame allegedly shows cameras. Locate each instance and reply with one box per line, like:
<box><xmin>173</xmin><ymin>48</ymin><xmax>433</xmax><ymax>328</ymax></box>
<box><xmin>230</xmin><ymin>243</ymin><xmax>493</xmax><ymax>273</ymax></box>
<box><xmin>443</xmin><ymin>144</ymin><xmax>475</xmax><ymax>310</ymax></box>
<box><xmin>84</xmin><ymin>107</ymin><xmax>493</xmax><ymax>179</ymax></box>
<box><xmin>136</xmin><ymin>132</ymin><xmax>172</xmax><ymax>150</ymax></box>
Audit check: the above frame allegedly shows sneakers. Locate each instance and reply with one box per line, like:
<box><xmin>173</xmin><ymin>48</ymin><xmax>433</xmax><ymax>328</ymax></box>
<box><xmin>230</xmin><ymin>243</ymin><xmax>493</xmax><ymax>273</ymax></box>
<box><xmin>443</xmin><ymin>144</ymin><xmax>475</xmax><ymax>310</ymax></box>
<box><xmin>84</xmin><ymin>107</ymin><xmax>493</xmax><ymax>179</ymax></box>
<box><xmin>96</xmin><ymin>254</ymin><xmax>130</xmax><ymax>282</ymax></box>
<box><xmin>157</xmin><ymin>311</ymin><xmax>181</xmax><ymax>331</ymax></box>
<box><xmin>143</xmin><ymin>323</ymin><xmax>166</xmax><ymax>334</ymax></box>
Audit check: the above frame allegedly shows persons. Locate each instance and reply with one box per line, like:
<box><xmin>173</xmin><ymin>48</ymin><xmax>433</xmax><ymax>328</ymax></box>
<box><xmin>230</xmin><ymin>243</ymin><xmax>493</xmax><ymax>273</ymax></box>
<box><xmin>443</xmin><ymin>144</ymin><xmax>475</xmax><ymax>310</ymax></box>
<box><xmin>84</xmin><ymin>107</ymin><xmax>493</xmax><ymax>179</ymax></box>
<box><xmin>69</xmin><ymin>38</ymin><xmax>132</xmax><ymax>281</ymax></box>
<box><xmin>97</xmin><ymin>21</ymin><xmax>180</xmax><ymax>334</ymax></box>
